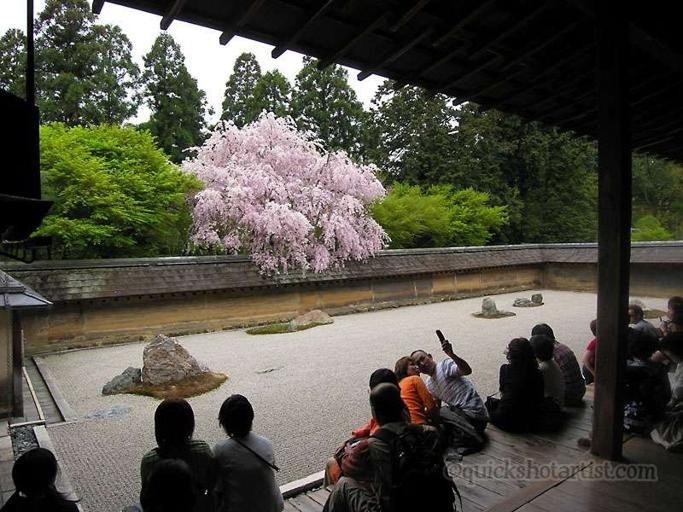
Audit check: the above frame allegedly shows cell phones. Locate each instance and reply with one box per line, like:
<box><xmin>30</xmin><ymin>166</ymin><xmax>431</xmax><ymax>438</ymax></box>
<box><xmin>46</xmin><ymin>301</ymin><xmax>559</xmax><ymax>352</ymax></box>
<box><xmin>436</xmin><ymin>330</ymin><xmax>445</xmax><ymax>344</ymax></box>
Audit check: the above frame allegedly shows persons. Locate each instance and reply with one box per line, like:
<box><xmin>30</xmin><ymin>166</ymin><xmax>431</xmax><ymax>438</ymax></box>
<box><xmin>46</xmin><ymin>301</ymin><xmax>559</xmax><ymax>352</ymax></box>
<box><xmin>325</xmin><ymin>295</ymin><xmax>681</xmax><ymax>510</ymax></box>
<box><xmin>139</xmin><ymin>398</ymin><xmax>216</xmax><ymax>512</ymax></box>
<box><xmin>0</xmin><ymin>449</ymin><xmax>78</xmax><ymax>511</ymax></box>
<box><xmin>214</xmin><ymin>395</ymin><xmax>285</xmax><ymax>511</ymax></box>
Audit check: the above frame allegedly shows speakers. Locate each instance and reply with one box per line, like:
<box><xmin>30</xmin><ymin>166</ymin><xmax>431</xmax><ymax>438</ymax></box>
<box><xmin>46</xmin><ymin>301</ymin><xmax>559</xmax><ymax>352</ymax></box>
<box><xmin>0</xmin><ymin>88</ymin><xmax>40</xmax><ymax>200</ymax></box>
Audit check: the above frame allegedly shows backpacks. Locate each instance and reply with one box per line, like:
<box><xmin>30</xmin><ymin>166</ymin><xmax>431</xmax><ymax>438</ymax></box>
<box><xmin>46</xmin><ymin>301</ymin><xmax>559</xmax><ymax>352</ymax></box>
<box><xmin>371</xmin><ymin>426</ymin><xmax>456</xmax><ymax>510</ymax></box>
<box><xmin>438</xmin><ymin>405</ymin><xmax>490</xmax><ymax>455</ymax></box>
